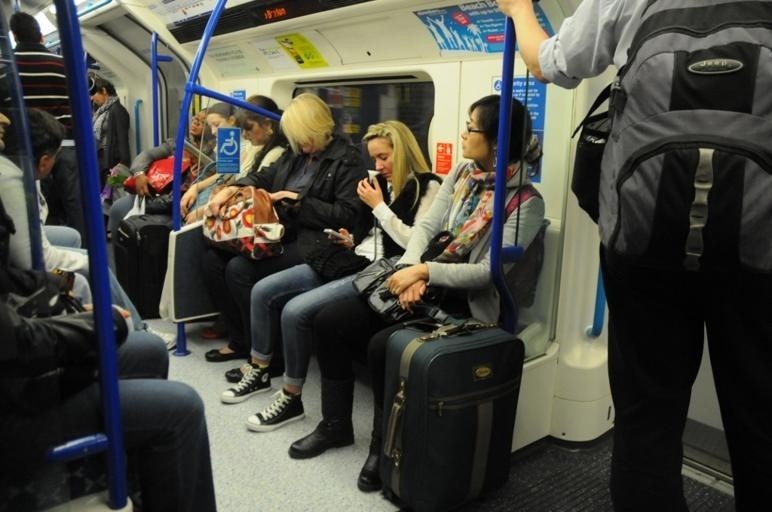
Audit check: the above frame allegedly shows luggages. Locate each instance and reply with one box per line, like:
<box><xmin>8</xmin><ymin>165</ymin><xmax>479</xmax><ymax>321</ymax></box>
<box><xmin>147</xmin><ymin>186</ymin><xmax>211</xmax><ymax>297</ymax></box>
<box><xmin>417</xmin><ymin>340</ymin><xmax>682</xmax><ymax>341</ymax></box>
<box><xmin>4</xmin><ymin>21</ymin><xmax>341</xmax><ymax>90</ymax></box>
<box><xmin>376</xmin><ymin>309</ymin><xmax>526</xmax><ymax>512</ymax></box>
<box><xmin>112</xmin><ymin>210</ymin><xmax>172</xmax><ymax>321</ymax></box>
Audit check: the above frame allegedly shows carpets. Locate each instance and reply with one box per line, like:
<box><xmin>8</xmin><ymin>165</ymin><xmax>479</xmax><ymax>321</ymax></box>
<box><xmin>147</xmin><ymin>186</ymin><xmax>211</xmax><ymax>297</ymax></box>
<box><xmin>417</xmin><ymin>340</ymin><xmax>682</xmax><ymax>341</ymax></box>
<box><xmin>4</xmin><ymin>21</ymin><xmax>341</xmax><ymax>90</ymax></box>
<box><xmin>458</xmin><ymin>433</ymin><xmax>736</xmax><ymax>512</ymax></box>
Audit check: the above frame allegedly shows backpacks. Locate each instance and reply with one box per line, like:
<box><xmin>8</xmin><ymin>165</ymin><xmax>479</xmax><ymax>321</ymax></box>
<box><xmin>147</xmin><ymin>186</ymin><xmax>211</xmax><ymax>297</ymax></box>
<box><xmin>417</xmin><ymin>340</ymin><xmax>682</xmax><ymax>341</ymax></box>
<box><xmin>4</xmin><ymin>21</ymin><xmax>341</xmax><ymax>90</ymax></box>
<box><xmin>569</xmin><ymin>0</ymin><xmax>772</xmax><ymax>332</ymax></box>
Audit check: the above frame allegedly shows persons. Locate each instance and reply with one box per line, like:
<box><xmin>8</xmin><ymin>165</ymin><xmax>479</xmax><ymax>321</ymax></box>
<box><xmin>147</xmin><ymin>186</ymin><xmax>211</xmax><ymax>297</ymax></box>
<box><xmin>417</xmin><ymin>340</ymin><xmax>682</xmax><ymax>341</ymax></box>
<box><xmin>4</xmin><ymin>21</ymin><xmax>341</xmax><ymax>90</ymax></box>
<box><xmin>0</xmin><ymin>210</ymin><xmax>216</xmax><ymax>510</ymax></box>
<box><xmin>222</xmin><ymin>122</ymin><xmax>444</xmax><ymax>433</ymax></box>
<box><xmin>204</xmin><ymin>94</ymin><xmax>368</xmax><ymax>383</ymax></box>
<box><xmin>288</xmin><ymin>94</ymin><xmax>545</xmax><ymax>491</ymax></box>
<box><xmin>2</xmin><ymin>14</ymin><xmax>285</xmax><ymax>340</ymax></box>
<box><xmin>496</xmin><ymin>0</ymin><xmax>771</xmax><ymax>509</ymax></box>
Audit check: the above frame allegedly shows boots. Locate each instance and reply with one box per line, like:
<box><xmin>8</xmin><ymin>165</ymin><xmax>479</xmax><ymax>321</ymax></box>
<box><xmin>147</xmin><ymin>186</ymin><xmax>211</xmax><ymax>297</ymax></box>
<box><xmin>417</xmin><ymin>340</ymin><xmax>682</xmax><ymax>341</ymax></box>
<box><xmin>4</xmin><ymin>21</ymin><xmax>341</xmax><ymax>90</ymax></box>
<box><xmin>355</xmin><ymin>387</ymin><xmax>398</xmax><ymax>493</ymax></box>
<box><xmin>286</xmin><ymin>374</ymin><xmax>357</xmax><ymax>462</ymax></box>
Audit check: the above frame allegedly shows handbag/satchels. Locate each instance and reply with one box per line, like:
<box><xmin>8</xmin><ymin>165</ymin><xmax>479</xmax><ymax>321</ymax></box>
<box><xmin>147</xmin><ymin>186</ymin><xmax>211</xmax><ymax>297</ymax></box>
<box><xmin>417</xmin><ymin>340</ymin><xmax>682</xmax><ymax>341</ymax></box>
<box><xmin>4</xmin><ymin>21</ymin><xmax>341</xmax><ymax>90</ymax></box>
<box><xmin>295</xmin><ymin>225</ymin><xmax>373</xmax><ymax>280</ymax></box>
<box><xmin>351</xmin><ymin>257</ymin><xmax>440</xmax><ymax>324</ymax></box>
<box><xmin>122</xmin><ymin>147</ymin><xmax>192</xmax><ymax>198</ymax></box>
<box><xmin>0</xmin><ymin>281</ymin><xmax>101</xmax><ymax>408</ymax></box>
<box><xmin>203</xmin><ymin>180</ymin><xmax>291</xmax><ymax>262</ymax></box>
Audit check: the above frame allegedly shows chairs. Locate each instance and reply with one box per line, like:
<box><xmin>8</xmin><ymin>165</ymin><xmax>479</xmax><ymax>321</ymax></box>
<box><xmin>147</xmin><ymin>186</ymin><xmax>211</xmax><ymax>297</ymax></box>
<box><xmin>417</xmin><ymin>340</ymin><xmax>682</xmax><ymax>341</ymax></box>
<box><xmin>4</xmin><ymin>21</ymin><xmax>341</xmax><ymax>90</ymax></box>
<box><xmin>495</xmin><ymin>216</ymin><xmax>552</xmax><ymax>360</ymax></box>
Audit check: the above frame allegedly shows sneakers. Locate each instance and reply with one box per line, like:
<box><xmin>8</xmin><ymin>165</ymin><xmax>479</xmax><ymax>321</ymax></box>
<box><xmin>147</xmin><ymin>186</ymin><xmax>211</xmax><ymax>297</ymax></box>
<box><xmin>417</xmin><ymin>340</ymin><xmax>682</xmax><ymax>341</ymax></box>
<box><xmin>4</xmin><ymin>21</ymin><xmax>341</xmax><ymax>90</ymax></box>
<box><xmin>143</xmin><ymin>327</ymin><xmax>178</xmax><ymax>351</ymax></box>
<box><xmin>220</xmin><ymin>363</ymin><xmax>273</xmax><ymax>404</ymax></box>
<box><xmin>244</xmin><ymin>388</ymin><xmax>307</xmax><ymax>434</ymax></box>
<box><xmin>200</xmin><ymin>327</ymin><xmax>223</xmax><ymax>340</ymax></box>
<box><xmin>203</xmin><ymin>346</ymin><xmax>249</xmax><ymax>363</ymax></box>
<box><xmin>224</xmin><ymin>366</ymin><xmax>243</xmax><ymax>384</ymax></box>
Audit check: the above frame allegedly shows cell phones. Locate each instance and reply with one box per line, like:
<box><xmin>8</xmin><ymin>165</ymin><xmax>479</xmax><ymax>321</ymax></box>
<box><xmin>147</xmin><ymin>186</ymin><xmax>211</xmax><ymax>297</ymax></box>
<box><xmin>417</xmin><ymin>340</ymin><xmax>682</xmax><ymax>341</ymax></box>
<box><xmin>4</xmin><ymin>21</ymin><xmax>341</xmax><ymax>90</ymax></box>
<box><xmin>323</xmin><ymin>228</ymin><xmax>353</xmax><ymax>245</ymax></box>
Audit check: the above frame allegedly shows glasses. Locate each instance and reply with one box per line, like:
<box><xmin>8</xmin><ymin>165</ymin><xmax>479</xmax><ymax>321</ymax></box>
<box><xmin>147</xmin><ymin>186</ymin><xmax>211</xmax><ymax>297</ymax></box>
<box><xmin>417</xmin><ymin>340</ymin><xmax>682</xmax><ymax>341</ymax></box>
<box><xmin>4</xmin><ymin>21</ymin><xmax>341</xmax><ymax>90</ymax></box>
<box><xmin>236</xmin><ymin>121</ymin><xmax>255</xmax><ymax>131</ymax></box>
<box><xmin>465</xmin><ymin>120</ymin><xmax>493</xmax><ymax>135</ymax></box>
<box><xmin>191</xmin><ymin>116</ymin><xmax>208</xmax><ymax>126</ymax></box>
